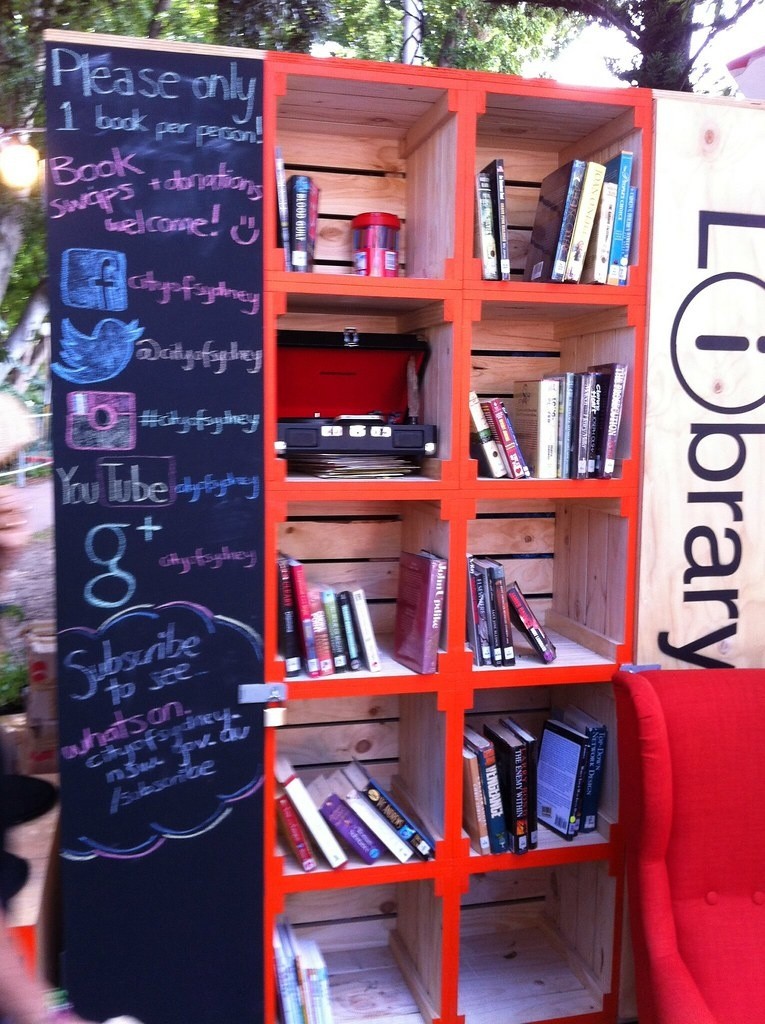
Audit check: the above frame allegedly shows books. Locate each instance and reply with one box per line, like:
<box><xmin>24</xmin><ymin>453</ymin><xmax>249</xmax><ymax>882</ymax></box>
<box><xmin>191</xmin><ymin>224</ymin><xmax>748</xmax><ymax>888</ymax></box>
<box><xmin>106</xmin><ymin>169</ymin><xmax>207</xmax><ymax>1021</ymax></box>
<box><xmin>271</xmin><ymin>149</ymin><xmax>635</xmax><ymax>1023</ymax></box>
<box><xmin>504</xmin><ymin>581</ymin><xmax>557</xmax><ymax>663</ymax></box>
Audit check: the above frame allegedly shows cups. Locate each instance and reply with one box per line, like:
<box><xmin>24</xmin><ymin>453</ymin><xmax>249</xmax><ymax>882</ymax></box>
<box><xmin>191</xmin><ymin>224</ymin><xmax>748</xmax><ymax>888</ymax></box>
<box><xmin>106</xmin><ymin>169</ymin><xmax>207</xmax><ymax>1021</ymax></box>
<box><xmin>352</xmin><ymin>212</ymin><xmax>400</xmax><ymax>277</ymax></box>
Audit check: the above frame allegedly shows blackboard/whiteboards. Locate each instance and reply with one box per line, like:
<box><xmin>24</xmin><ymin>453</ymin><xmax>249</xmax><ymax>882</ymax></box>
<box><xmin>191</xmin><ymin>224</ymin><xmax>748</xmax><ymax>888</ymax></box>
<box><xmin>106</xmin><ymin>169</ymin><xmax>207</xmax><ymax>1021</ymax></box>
<box><xmin>40</xmin><ymin>24</ymin><xmax>269</xmax><ymax>1024</ymax></box>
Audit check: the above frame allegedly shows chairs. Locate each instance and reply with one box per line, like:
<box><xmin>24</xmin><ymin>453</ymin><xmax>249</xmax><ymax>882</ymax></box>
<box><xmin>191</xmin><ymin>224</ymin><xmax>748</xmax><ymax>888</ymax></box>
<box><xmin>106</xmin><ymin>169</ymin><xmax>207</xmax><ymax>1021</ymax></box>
<box><xmin>613</xmin><ymin>667</ymin><xmax>765</xmax><ymax>1024</ymax></box>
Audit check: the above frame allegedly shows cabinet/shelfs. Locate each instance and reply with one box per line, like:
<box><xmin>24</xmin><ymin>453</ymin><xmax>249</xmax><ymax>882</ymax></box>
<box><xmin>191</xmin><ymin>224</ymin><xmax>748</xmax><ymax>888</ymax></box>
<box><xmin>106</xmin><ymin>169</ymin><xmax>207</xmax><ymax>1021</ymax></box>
<box><xmin>264</xmin><ymin>51</ymin><xmax>656</xmax><ymax>1016</ymax></box>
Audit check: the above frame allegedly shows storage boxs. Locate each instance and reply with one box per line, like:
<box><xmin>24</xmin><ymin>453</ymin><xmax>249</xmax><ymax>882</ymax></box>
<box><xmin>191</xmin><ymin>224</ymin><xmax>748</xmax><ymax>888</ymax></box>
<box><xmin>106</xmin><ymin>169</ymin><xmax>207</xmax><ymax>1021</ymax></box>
<box><xmin>0</xmin><ymin>619</ymin><xmax>56</xmax><ymax>775</ymax></box>
